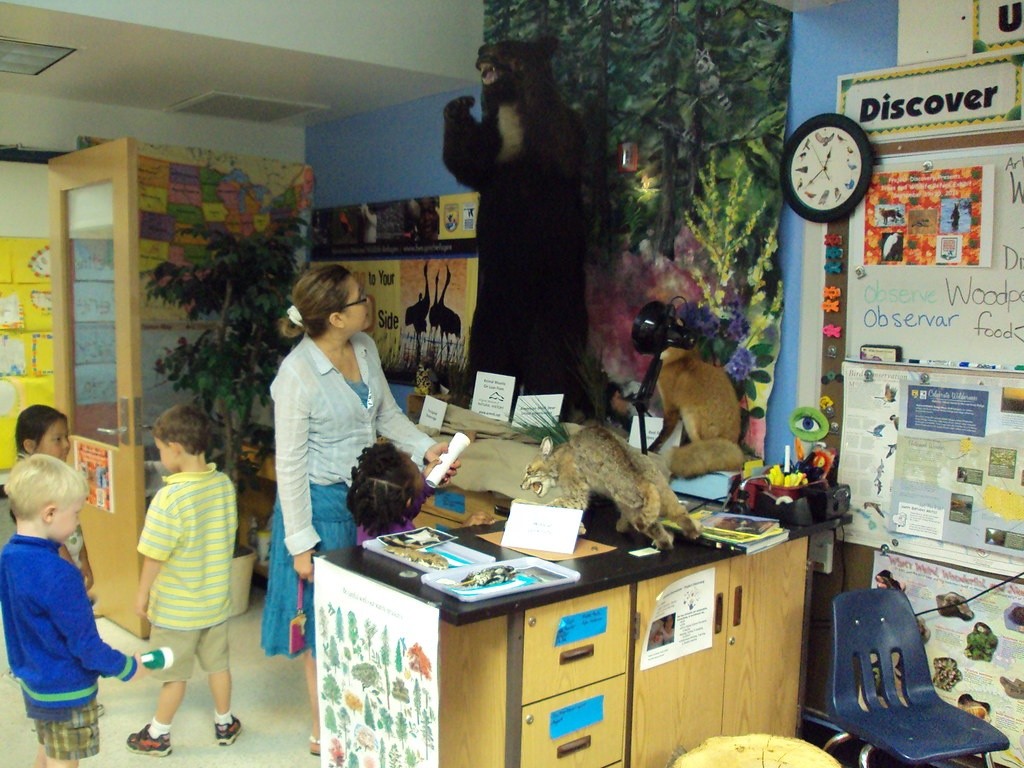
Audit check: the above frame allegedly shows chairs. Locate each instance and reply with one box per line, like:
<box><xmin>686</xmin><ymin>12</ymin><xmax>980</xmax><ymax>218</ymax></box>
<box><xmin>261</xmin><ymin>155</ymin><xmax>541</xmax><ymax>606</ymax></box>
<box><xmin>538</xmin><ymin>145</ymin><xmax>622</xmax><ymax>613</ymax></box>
<box><xmin>822</xmin><ymin>585</ymin><xmax>1009</xmax><ymax>768</ymax></box>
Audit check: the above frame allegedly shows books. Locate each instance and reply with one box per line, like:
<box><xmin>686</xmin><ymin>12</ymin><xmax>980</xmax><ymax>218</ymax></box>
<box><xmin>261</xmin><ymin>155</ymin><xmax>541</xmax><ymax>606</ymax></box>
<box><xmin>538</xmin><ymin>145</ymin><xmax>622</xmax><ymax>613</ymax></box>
<box><xmin>659</xmin><ymin>509</ymin><xmax>789</xmax><ymax>555</ymax></box>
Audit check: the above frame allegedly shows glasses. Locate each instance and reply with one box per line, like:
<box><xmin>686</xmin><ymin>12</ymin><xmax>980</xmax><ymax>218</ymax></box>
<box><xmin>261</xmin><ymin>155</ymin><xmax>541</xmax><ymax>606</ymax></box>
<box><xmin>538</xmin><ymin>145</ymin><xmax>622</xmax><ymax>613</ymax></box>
<box><xmin>331</xmin><ymin>290</ymin><xmax>366</xmax><ymax>312</ymax></box>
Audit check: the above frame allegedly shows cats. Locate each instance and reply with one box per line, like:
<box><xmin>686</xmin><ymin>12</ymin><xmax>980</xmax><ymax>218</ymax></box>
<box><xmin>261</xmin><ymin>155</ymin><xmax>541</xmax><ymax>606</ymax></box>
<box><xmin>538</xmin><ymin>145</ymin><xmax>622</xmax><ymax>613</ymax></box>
<box><xmin>519</xmin><ymin>425</ymin><xmax>696</xmax><ymax>551</ymax></box>
<box><xmin>647</xmin><ymin>334</ymin><xmax>744</xmax><ymax>478</ymax></box>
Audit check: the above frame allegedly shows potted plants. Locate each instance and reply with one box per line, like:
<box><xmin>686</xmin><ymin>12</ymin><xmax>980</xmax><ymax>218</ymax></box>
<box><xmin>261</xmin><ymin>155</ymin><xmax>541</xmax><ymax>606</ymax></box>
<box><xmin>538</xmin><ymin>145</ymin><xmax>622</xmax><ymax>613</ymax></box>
<box><xmin>143</xmin><ymin>202</ymin><xmax>332</xmax><ymax>621</ymax></box>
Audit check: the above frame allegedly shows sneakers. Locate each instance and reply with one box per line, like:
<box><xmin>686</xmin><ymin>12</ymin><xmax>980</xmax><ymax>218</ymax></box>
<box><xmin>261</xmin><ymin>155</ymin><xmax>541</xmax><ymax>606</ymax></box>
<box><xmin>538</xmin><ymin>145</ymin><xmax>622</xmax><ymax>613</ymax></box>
<box><xmin>214</xmin><ymin>715</ymin><xmax>241</xmax><ymax>746</ymax></box>
<box><xmin>126</xmin><ymin>724</ymin><xmax>172</xmax><ymax>756</ymax></box>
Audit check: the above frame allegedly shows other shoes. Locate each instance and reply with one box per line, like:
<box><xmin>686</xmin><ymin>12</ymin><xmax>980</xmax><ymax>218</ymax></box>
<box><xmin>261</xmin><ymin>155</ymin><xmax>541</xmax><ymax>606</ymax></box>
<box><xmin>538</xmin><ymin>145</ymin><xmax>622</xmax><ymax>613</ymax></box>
<box><xmin>310</xmin><ymin>738</ymin><xmax>320</xmax><ymax>755</ymax></box>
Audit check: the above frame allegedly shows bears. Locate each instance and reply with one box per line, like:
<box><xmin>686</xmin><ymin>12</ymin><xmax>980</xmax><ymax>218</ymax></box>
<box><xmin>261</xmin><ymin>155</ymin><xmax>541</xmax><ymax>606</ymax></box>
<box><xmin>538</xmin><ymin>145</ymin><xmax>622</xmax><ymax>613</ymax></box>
<box><xmin>441</xmin><ymin>40</ymin><xmax>591</xmax><ymax>417</ymax></box>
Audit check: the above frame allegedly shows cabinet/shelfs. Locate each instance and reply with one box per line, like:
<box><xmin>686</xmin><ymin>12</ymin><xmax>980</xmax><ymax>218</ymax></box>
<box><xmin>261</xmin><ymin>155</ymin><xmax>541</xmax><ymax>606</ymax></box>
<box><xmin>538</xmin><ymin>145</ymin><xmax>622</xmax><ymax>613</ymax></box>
<box><xmin>436</xmin><ymin>527</ymin><xmax>836</xmax><ymax>767</ymax></box>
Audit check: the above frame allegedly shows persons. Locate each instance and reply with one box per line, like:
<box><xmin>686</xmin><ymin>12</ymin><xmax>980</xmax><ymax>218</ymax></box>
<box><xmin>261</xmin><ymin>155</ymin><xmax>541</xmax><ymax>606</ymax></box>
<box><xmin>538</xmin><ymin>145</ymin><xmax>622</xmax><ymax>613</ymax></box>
<box><xmin>124</xmin><ymin>405</ymin><xmax>243</xmax><ymax>756</ymax></box>
<box><xmin>8</xmin><ymin>404</ymin><xmax>94</xmax><ymax>591</ymax></box>
<box><xmin>259</xmin><ymin>264</ymin><xmax>462</xmax><ymax>758</ymax></box>
<box><xmin>0</xmin><ymin>454</ymin><xmax>149</xmax><ymax>768</ymax></box>
<box><xmin>346</xmin><ymin>443</ymin><xmax>496</xmax><ymax>546</ymax></box>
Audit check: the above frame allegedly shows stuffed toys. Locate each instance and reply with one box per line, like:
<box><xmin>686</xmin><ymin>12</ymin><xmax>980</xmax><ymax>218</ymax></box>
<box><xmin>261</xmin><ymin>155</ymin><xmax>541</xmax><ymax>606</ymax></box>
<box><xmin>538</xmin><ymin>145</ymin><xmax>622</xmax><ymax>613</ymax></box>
<box><xmin>647</xmin><ymin>346</ymin><xmax>744</xmax><ymax>477</ymax></box>
<box><xmin>441</xmin><ymin>36</ymin><xmax>589</xmax><ymax>409</ymax></box>
<box><xmin>520</xmin><ymin>426</ymin><xmax>701</xmax><ymax>551</ymax></box>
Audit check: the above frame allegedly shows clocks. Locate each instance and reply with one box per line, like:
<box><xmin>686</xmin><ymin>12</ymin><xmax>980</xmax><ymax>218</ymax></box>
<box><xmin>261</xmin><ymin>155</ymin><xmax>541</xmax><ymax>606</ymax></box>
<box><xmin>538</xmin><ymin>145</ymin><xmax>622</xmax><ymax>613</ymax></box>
<box><xmin>780</xmin><ymin>112</ymin><xmax>875</xmax><ymax>222</ymax></box>
